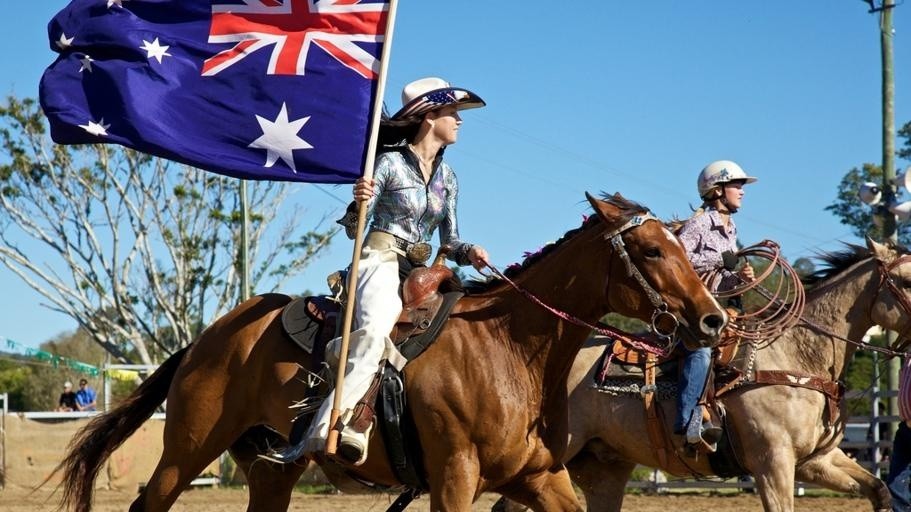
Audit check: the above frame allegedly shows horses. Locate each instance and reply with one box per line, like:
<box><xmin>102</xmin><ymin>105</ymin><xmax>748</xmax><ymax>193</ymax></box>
<box><xmin>492</xmin><ymin>231</ymin><xmax>911</xmax><ymax>512</ymax></box>
<box><xmin>28</xmin><ymin>190</ymin><xmax>731</xmax><ymax>512</ymax></box>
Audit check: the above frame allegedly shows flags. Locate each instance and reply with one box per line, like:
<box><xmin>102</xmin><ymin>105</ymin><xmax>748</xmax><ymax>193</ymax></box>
<box><xmin>42</xmin><ymin>0</ymin><xmax>392</xmax><ymax>183</ymax></box>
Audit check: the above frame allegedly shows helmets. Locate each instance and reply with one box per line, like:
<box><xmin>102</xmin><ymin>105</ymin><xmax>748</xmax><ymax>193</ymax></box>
<box><xmin>697</xmin><ymin>159</ymin><xmax>759</xmax><ymax>199</ymax></box>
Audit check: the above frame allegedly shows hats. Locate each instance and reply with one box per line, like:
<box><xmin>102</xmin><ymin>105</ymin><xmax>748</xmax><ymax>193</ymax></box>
<box><xmin>389</xmin><ymin>76</ymin><xmax>487</xmax><ymax>121</ymax></box>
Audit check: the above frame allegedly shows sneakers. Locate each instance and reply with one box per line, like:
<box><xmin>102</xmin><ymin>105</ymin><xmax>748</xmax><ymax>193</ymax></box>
<box><xmin>339</xmin><ymin>408</ymin><xmax>367</xmax><ymax>463</ymax></box>
<box><xmin>683</xmin><ymin>421</ymin><xmax>723</xmax><ymax>459</ymax></box>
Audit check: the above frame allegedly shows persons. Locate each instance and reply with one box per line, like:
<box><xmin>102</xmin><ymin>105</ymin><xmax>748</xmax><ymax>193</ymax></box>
<box><xmin>666</xmin><ymin>159</ymin><xmax>757</xmax><ymax>442</ymax></box>
<box><xmin>53</xmin><ymin>380</ymin><xmax>79</xmax><ymax>412</ymax></box>
<box><xmin>304</xmin><ymin>77</ymin><xmax>490</xmax><ymax>459</ymax></box>
<box><xmin>74</xmin><ymin>379</ymin><xmax>100</xmax><ymax>411</ymax></box>
